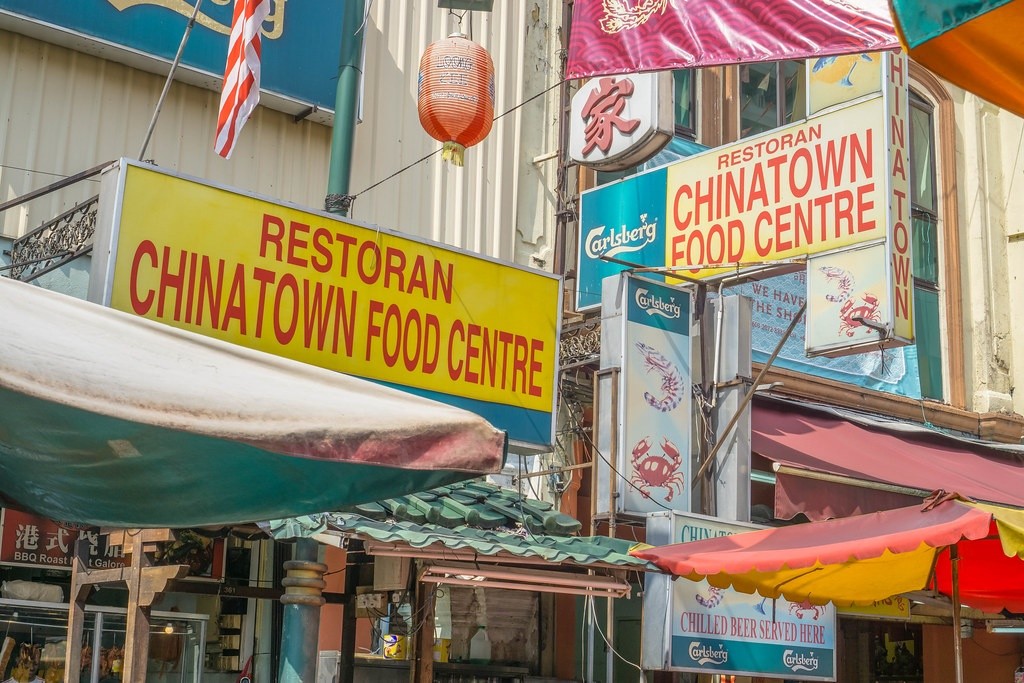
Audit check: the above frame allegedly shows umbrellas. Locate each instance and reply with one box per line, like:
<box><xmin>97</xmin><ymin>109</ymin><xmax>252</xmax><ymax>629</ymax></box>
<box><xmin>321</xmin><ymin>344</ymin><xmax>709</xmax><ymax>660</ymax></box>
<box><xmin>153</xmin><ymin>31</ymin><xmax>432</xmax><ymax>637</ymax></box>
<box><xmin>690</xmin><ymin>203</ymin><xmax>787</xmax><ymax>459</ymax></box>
<box><xmin>628</xmin><ymin>490</ymin><xmax>1024</xmax><ymax>683</ymax></box>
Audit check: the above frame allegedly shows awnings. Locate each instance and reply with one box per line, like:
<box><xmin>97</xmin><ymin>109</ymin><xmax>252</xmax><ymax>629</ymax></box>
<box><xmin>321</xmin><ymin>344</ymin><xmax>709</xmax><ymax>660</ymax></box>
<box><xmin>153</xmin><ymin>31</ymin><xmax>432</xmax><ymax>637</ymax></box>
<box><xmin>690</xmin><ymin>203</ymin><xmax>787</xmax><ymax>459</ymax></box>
<box><xmin>0</xmin><ymin>271</ymin><xmax>511</xmax><ymax>533</ymax></box>
<box><xmin>753</xmin><ymin>403</ymin><xmax>1024</xmax><ymax>526</ymax></box>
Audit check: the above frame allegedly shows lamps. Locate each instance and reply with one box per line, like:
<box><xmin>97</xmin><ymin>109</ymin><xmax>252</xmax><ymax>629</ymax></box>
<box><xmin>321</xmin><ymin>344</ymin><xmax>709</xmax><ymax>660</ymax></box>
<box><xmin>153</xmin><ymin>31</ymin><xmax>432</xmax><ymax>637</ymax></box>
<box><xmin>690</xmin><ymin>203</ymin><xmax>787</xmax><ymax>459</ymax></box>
<box><xmin>748</xmin><ymin>468</ymin><xmax>779</xmax><ymax>485</ymax></box>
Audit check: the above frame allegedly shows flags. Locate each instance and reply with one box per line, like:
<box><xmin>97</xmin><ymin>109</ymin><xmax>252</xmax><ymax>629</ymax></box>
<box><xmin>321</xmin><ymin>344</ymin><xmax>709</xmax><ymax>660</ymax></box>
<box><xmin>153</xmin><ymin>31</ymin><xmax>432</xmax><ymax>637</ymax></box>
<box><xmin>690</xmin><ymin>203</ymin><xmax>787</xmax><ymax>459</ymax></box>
<box><xmin>214</xmin><ymin>0</ymin><xmax>269</xmax><ymax>161</ymax></box>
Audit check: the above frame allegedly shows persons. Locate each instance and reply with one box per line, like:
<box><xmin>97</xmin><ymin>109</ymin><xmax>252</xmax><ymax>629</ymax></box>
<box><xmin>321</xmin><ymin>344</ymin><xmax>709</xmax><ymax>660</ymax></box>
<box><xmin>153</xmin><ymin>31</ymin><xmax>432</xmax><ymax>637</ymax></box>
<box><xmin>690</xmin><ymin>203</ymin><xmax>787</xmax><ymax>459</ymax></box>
<box><xmin>1</xmin><ymin>647</ymin><xmax>46</xmax><ymax>683</ymax></box>
